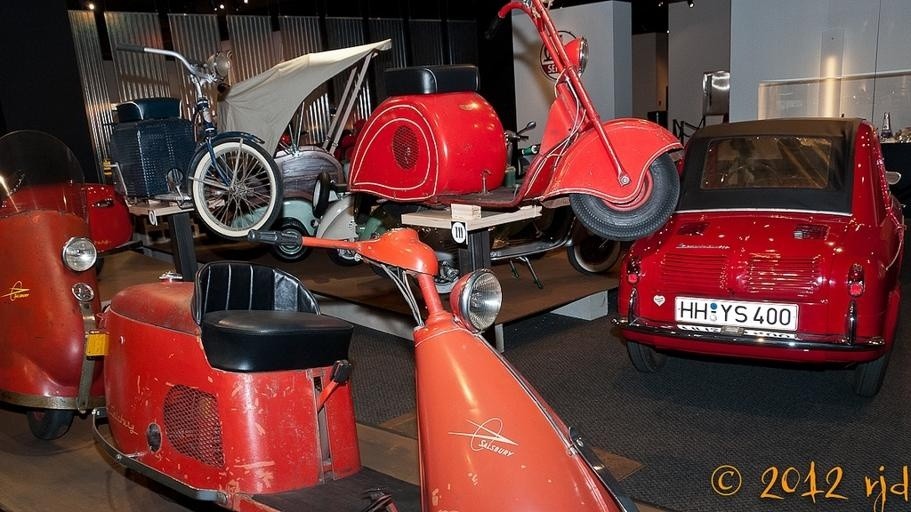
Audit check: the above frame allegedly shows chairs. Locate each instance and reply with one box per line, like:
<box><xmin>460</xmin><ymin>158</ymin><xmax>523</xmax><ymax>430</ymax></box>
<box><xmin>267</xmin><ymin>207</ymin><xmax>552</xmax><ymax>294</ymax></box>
<box><xmin>190</xmin><ymin>259</ymin><xmax>358</xmax><ymax>370</ymax></box>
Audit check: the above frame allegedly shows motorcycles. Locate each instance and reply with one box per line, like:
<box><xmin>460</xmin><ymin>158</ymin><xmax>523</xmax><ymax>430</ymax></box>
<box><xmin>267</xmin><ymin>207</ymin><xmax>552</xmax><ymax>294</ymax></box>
<box><xmin>0</xmin><ymin>128</ymin><xmax>131</xmax><ymax>446</ymax></box>
<box><xmin>84</xmin><ymin>226</ymin><xmax>639</xmax><ymax>511</ymax></box>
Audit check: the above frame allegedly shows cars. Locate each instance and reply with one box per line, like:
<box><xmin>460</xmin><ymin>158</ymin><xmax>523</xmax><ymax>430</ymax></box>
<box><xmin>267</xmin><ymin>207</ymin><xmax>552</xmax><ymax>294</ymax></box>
<box><xmin>613</xmin><ymin>115</ymin><xmax>911</xmax><ymax>398</ymax></box>
<box><xmin>0</xmin><ymin>182</ymin><xmax>133</xmax><ymax>276</ymax></box>
